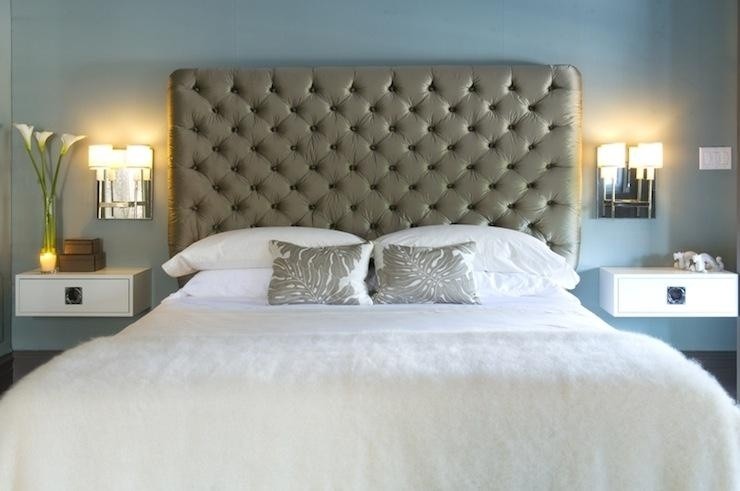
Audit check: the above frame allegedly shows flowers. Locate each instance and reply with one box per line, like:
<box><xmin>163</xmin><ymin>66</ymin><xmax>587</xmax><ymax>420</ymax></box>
<box><xmin>13</xmin><ymin>121</ymin><xmax>85</xmax><ymax>255</ymax></box>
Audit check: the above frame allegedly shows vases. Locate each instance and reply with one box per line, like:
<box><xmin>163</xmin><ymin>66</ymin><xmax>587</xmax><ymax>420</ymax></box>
<box><xmin>37</xmin><ymin>197</ymin><xmax>61</xmax><ymax>273</ymax></box>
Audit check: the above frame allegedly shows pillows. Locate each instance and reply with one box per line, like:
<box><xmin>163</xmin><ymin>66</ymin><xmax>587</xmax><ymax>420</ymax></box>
<box><xmin>376</xmin><ymin>241</ymin><xmax>482</xmax><ymax>303</ymax></box>
<box><xmin>375</xmin><ymin>226</ymin><xmax>571</xmax><ymax>272</ymax></box>
<box><xmin>162</xmin><ymin>226</ymin><xmax>367</xmax><ymax>278</ymax></box>
<box><xmin>267</xmin><ymin>239</ymin><xmax>376</xmax><ymax>305</ymax></box>
<box><xmin>477</xmin><ymin>271</ymin><xmax>579</xmax><ymax>300</ymax></box>
<box><xmin>161</xmin><ymin>270</ymin><xmax>272</xmax><ymax>304</ymax></box>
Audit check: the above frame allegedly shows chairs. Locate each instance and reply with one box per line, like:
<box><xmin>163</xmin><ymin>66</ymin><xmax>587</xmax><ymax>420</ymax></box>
<box><xmin>597</xmin><ymin>266</ymin><xmax>738</xmax><ymax>316</ymax></box>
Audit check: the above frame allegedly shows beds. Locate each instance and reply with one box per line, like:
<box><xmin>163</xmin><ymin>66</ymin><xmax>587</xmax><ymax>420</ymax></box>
<box><xmin>0</xmin><ymin>64</ymin><xmax>739</xmax><ymax>491</ymax></box>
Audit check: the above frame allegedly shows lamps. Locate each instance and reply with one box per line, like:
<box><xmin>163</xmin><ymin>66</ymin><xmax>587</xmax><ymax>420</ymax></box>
<box><xmin>87</xmin><ymin>143</ymin><xmax>153</xmax><ymax>221</ymax></box>
<box><xmin>596</xmin><ymin>142</ymin><xmax>664</xmax><ymax>219</ymax></box>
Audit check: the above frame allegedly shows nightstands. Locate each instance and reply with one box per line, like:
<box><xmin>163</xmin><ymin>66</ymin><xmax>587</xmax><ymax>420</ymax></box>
<box><xmin>15</xmin><ymin>266</ymin><xmax>151</xmax><ymax>318</ymax></box>
